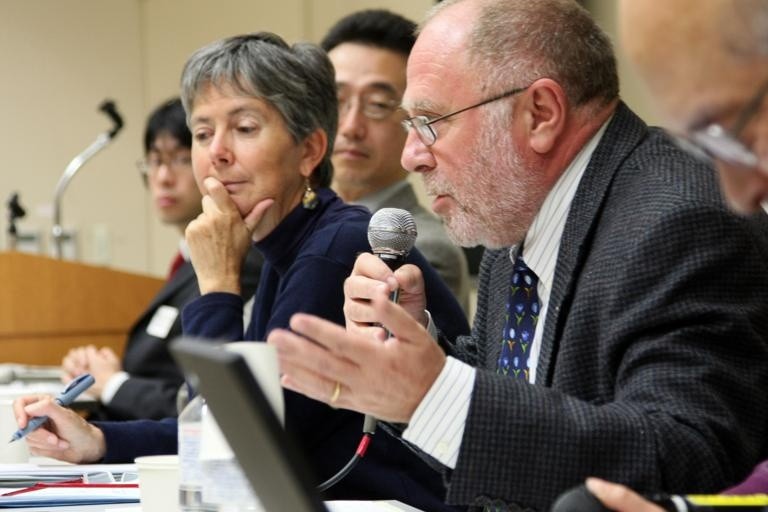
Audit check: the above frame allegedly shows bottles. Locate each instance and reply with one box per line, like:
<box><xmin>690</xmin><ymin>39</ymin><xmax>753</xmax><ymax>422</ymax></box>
<box><xmin>201</xmin><ymin>341</ymin><xmax>284</xmax><ymax>511</ymax></box>
<box><xmin>178</xmin><ymin>390</ymin><xmax>233</xmax><ymax>512</ymax></box>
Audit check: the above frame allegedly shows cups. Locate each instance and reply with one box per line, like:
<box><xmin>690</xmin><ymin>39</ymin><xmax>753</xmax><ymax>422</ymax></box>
<box><xmin>134</xmin><ymin>455</ymin><xmax>179</xmax><ymax>512</ymax></box>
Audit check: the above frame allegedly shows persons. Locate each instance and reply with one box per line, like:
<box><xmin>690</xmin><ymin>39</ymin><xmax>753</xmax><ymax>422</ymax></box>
<box><xmin>266</xmin><ymin>0</ymin><xmax>767</xmax><ymax>511</ymax></box>
<box><xmin>59</xmin><ymin>97</ymin><xmax>204</xmax><ymax>421</ymax></box>
<box><xmin>14</xmin><ymin>31</ymin><xmax>474</xmax><ymax>509</ymax></box>
<box><xmin>584</xmin><ymin>0</ymin><xmax>768</xmax><ymax>512</ymax></box>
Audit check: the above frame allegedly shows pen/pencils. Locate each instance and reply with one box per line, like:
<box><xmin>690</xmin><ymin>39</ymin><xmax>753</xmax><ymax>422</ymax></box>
<box><xmin>6</xmin><ymin>374</ymin><xmax>95</xmax><ymax>445</ymax></box>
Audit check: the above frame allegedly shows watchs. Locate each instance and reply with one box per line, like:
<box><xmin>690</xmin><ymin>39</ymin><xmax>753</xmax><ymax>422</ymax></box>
<box><xmin>319</xmin><ymin>9</ymin><xmax>471</xmax><ymax>321</ymax></box>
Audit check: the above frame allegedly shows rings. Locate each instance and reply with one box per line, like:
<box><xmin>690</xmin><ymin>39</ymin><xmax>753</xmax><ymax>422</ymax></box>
<box><xmin>329</xmin><ymin>382</ymin><xmax>341</xmax><ymax>402</ymax></box>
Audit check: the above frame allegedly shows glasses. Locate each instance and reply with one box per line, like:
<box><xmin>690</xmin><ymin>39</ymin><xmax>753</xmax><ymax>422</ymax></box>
<box><xmin>136</xmin><ymin>151</ymin><xmax>191</xmax><ymax>177</ymax></box>
<box><xmin>664</xmin><ymin>78</ymin><xmax>767</xmax><ymax>169</ymax></box>
<box><xmin>335</xmin><ymin>89</ymin><xmax>401</xmax><ymax>121</ymax></box>
<box><xmin>401</xmin><ymin>86</ymin><xmax>527</xmax><ymax>146</ymax></box>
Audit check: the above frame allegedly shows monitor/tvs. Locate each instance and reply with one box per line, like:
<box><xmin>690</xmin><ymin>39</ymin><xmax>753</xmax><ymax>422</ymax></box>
<box><xmin>167</xmin><ymin>337</ymin><xmax>330</xmax><ymax>512</ymax></box>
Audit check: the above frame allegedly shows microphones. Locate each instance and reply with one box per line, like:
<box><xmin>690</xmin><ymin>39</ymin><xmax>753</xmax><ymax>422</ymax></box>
<box><xmin>554</xmin><ymin>485</ymin><xmax>768</xmax><ymax>512</ymax></box>
<box><xmin>45</xmin><ymin>99</ymin><xmax>124</xmax><ymax>257</ymax></box>
<box><xmin>360</xmin><ymin>207</ymin><xmax>416</xmax><ymax>436</ymax></box>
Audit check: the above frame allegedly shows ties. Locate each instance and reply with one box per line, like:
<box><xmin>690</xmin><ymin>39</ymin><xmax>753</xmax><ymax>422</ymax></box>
<box><xmin>497</xmin><ymin>240</ymin><xmax>543</xmax><ymax>382</ymax></box>
<box><xmin>168</xmin><ymin>253</ymin><xmax>187</xmax><ymax>281</ymax></box>
